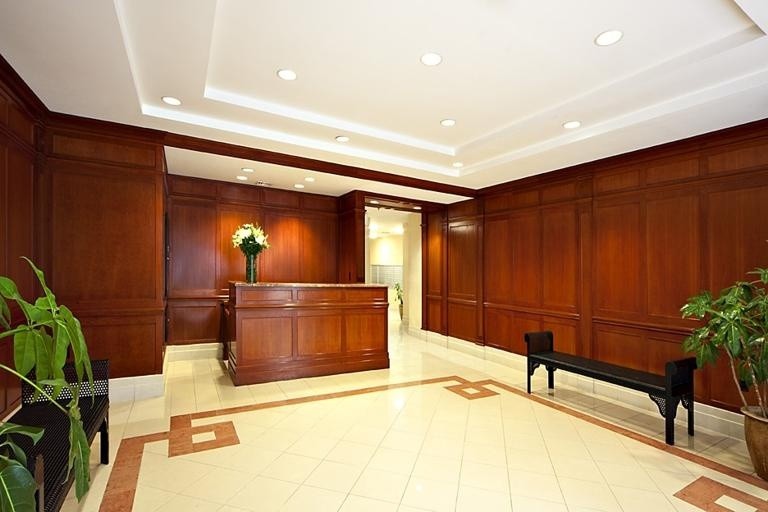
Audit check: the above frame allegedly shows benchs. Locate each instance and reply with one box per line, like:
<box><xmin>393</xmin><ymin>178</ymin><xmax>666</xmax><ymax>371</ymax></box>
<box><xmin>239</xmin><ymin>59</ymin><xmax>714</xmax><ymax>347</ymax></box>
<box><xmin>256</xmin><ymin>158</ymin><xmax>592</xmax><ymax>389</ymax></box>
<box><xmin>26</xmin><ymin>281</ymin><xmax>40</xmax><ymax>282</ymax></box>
<box><xmin>1</xmin><ymin>359</ymin><xmax>109</xmax><ymax>512</ymax></box>
<box><xmin>524</xmin><ymin>330</ymin><xmax>698</xmax><ymax>446</ymax></box>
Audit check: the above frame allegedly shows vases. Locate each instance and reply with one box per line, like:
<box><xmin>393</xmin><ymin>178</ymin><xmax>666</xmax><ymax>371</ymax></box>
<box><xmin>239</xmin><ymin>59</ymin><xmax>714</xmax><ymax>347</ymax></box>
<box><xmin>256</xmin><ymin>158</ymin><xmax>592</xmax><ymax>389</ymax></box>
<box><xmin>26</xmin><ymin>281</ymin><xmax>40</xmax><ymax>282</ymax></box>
<box><xmin>240</xmin><ymin>243</ymin><xmax>262</xmax><ymax>282</ymax></box>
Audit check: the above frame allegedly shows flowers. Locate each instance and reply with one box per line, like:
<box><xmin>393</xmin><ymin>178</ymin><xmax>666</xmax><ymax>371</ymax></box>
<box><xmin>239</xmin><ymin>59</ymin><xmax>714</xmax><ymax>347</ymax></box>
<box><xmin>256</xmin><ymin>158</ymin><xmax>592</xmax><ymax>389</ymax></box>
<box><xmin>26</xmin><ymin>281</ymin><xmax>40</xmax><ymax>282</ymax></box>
<box><xmin>231</xmin><ymin>221</ymin><xmax>272</xmax><ymax>256</ymax></box>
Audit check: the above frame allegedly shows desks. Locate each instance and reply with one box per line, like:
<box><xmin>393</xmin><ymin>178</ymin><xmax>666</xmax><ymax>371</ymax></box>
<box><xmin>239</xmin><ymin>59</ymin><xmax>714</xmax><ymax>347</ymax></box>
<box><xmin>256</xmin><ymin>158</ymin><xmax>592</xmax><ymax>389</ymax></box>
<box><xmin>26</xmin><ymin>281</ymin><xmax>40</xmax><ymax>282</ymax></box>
<box><xmin>221</xmin><ymin>279</ymin><xmax>390</xmax><ymax>387</ymax></box>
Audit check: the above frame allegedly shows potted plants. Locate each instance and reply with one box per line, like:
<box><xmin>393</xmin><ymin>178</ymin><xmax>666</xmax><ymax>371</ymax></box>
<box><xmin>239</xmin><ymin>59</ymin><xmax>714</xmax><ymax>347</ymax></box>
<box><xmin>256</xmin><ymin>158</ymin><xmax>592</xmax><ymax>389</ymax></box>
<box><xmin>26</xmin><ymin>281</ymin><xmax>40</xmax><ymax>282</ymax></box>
<box><xmin>679</xmin><ymin>266</ymin><xmax>768</xmax><ymax>482</ymax></box>
<box><xmin>394</xmin><ymin>282</ymin><xmax>403</xmax><ymax>319</ymax></box>
